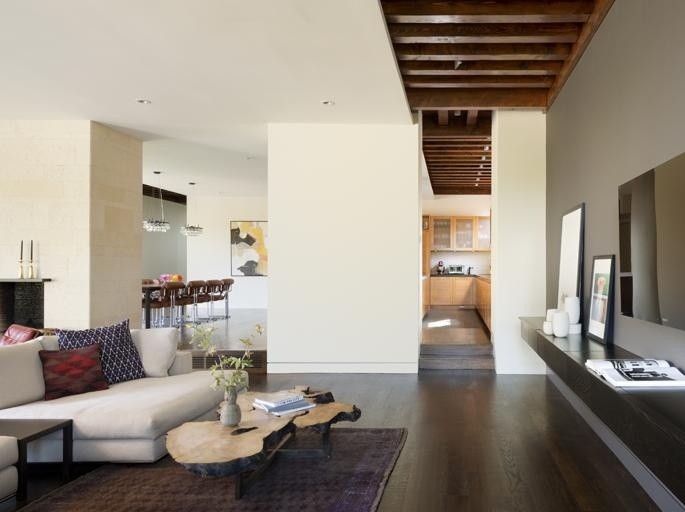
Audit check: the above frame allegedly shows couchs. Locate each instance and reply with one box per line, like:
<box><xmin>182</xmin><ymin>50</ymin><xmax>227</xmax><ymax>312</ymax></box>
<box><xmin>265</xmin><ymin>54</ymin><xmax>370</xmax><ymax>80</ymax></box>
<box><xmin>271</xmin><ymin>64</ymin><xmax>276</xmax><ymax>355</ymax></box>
<box><xmin>0</xmin><ymin>324</ymin><xmax>249</xmax><ymax>462</ymax></box>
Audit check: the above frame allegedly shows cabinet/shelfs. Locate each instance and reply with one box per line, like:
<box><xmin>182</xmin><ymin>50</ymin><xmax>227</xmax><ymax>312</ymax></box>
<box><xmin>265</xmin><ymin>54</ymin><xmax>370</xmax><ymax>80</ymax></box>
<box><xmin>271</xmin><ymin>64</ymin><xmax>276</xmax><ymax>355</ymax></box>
<box><xmin>423</xmin><ymin>214</ymin><xmax>491</xmax><ymax>332</ymax></box>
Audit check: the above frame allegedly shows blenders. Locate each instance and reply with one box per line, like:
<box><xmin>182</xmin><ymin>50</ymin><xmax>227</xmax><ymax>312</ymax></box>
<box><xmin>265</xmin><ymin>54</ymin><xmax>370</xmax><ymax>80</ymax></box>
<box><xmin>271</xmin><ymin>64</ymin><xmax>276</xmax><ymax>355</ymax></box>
<box><xmin>437</xmin><ymin>261</ymin><xmax>445</xmax><ymax>274</ymax></box>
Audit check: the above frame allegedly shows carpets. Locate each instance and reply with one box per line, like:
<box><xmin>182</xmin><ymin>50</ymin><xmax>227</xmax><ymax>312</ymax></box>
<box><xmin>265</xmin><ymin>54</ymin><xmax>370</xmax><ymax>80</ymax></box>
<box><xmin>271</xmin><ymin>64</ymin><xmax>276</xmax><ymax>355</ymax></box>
<box><xmin>17</xmin><ymin>427</ymin><xmax>407</xmax><ymax>512</ymax></box>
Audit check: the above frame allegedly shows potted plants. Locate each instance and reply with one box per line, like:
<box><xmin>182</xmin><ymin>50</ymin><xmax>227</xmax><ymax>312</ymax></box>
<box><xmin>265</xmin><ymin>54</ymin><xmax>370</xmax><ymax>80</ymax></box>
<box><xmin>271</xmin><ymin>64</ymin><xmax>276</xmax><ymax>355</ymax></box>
<box><xmin>184</xmin><ymin>324</ymin><xmax>265</xmax><ymax>428</ymax></box>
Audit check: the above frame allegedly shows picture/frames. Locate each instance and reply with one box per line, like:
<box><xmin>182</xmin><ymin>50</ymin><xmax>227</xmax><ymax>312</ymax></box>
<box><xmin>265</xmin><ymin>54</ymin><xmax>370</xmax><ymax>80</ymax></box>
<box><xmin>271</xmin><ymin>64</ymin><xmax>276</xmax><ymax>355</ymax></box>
<box><xmin>585</xmin><ymin>254</ymin><xmax>615</xmax><ymax>347</ymax></box>
<box><xmin>228</xmin><ymin>217</ymin><xmax>268</xmax><ymax>278</ymax></box>
<box><xmin>557</xmin><ymin>201</ymin><xmax>585</xmax><ymax>308</ymax></box>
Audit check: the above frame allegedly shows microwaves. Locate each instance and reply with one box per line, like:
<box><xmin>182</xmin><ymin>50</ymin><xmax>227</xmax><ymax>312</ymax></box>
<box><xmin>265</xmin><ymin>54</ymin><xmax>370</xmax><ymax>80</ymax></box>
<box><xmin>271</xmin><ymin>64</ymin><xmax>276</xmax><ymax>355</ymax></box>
<box><xmin>448</xmin><ymin>265</ymin><xmax>466</xmax><ymax>274</ymax></box>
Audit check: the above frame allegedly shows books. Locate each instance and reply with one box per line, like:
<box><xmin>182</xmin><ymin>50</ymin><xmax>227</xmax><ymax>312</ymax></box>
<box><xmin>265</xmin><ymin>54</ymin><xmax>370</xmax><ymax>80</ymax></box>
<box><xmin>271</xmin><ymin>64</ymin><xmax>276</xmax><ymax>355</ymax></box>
<box><xmin>253</xmin><ymin>389</ymin><xmax>306</xmax><ymax>406</ymax></box>
<box><xmin>252</xmin><ymin>400</ymin><xmax>317</xmax><ymax>415</ymax></box>
<box><xmin>583</xmin><ymin>358</ymin><xmax>685</xmax><ymax>389</ymax></box>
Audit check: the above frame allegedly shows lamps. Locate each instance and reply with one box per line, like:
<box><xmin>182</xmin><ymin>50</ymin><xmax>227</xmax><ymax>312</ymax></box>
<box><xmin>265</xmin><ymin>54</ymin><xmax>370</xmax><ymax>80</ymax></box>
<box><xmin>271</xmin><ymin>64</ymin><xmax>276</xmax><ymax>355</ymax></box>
<box><xmin>143</xmin><ymin>172</ymin><xmax>204</xmax><ymax>236</ymax></box>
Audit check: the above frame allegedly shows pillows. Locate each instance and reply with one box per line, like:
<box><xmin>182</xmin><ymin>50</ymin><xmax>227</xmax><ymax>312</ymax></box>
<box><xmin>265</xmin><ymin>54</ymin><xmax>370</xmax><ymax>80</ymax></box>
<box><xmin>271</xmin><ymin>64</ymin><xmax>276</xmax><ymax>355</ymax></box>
<box><xmin>37</xmin><ymin>318</ymin><xmax>147</xmax><ymax>401</ymax></box>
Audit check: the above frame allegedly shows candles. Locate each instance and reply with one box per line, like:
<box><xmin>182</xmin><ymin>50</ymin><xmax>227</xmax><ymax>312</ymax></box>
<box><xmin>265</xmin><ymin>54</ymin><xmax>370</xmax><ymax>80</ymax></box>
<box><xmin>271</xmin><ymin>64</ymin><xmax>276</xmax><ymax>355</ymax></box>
<box><xmin>20</xmin><ymin>239</ymin><xmax>34</xmax><ymax>263</ymax></box>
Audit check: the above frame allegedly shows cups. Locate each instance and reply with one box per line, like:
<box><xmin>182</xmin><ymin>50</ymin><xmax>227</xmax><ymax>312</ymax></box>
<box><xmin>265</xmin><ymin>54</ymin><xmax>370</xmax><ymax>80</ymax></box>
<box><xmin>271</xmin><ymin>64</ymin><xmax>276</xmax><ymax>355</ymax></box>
<box><xmin>544</xmin><ymin>297</ymin><xmax>582</xmax><ymax>337</ymax></box>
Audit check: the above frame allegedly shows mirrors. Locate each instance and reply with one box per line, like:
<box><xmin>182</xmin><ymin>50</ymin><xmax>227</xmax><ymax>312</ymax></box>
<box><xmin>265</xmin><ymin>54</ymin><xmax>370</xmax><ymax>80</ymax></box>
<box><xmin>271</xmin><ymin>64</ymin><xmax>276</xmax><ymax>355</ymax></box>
<box><xmin>617</xmin><ymin>152</ymin><xmax>685</xmax><ymax>331</ymax></box>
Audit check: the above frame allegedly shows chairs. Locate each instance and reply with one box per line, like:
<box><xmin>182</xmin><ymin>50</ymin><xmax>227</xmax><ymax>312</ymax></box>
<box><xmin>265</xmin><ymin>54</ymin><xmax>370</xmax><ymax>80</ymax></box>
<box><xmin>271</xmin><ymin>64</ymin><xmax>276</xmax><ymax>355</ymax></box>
<box><xmin>142</xmin><ymin>279</ymin><xmax>233</xmax><ymax>327</ymax></box>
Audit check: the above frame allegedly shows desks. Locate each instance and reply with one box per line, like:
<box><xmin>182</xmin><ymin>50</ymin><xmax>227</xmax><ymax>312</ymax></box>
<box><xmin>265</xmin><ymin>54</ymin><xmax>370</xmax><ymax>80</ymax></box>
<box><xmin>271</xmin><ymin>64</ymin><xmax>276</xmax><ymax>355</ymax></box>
<box><xmin>0</xmin><ymin>418</ymin><xmax>74</xmax><ymax>511</ymax></box>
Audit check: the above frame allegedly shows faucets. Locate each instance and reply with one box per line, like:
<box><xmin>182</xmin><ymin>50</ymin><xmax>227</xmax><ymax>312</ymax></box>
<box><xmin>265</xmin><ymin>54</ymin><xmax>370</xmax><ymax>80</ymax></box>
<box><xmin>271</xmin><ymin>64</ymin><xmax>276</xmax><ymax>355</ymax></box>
<box><xmin>468</xmin><ymin>266</ymin><xmax>474</xmax><ymax>274</ymax></box>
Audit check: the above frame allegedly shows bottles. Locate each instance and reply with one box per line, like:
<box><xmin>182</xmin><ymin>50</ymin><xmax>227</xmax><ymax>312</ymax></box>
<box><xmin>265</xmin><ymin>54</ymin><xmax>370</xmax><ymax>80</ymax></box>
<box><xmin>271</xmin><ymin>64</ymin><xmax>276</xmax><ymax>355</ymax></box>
<box><xmin>469</xmin><ymin>267</ymin><xmax>473</xmax><ymax>275</ymax></box>
